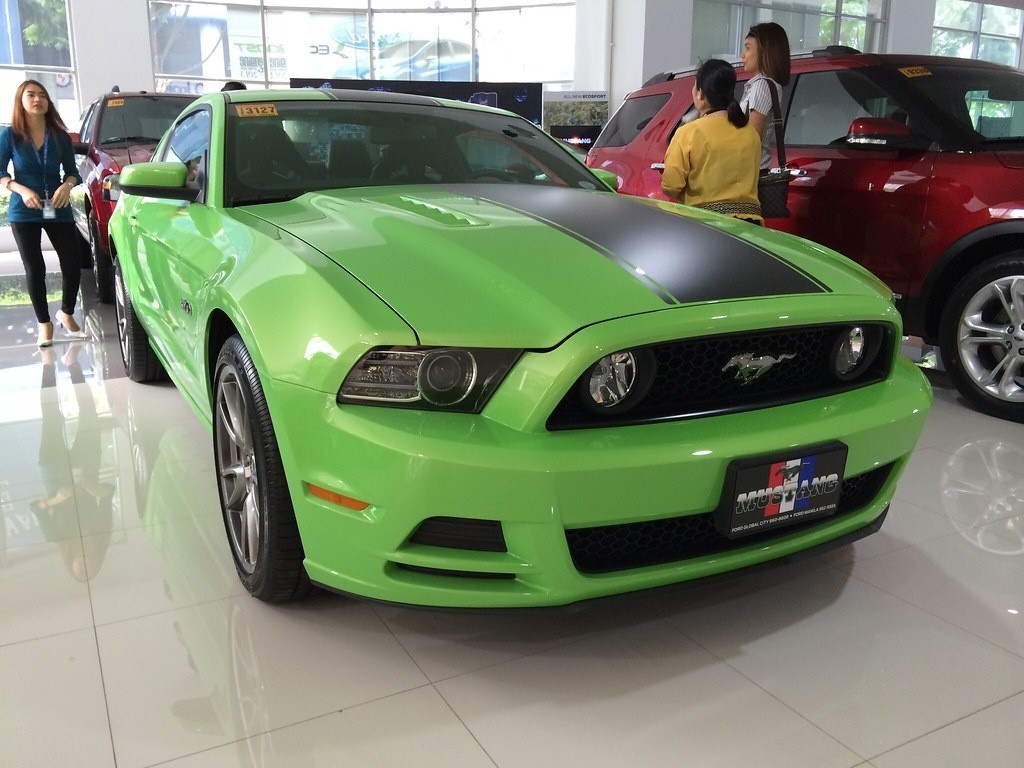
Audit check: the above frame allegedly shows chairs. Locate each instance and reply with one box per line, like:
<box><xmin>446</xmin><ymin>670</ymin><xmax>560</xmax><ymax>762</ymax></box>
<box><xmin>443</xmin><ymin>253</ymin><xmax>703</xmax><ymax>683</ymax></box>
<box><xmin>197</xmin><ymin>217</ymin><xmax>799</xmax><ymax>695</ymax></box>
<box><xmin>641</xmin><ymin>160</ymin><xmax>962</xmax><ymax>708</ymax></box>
<box><xmin>802</xmin><ymin>103</ymin><xmax>909</xmax><ymax>145</ymax></box>
<box><xmin>227</xmin><ymin>122</ymin><xmax>466</xmax><ymax>187</ymax></box>
<box><xmin>106</xmin><ymin>116</ymin><xmax>144</xmax><ymax>140</ymax></box>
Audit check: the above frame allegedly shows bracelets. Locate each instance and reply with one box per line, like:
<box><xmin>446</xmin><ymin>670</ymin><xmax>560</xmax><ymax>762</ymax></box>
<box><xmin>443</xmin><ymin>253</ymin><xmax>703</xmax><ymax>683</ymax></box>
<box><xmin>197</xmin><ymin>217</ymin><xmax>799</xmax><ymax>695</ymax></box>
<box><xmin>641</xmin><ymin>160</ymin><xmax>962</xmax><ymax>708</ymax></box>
<box><xmin>69</xmin><ymin>182</ymin><xmax>74</xmax><ymax>187</ymax></box>
<box><xmin>6</xmin><ymin>180</ymin><xmax>13</xmax><ymax>191</ymax></box>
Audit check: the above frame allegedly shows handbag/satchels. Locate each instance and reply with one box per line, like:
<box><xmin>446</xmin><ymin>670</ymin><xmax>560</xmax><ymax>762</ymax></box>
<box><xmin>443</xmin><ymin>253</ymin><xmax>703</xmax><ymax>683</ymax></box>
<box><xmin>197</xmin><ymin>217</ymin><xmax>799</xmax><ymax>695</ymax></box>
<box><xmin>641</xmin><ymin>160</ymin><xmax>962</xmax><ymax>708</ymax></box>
<box><xmin>745</xmin><ymin>78</ymin><xmax>792</xmax><ymax>218</ymax></box>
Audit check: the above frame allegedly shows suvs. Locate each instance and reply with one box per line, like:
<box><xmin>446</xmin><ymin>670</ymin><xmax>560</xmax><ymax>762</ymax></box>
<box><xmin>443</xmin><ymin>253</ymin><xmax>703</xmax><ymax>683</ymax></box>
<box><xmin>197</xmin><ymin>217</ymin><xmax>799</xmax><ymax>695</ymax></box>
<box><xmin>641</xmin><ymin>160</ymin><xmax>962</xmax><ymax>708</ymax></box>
<box><xmin>62</xmin><ymin>84</ymin><xmax>205</xmax><ymax>304</ymax></box>
<box><xmin>584</xmin><ymin>43</ymin><xmax>1024</xmax><ymax>423</ymax></box>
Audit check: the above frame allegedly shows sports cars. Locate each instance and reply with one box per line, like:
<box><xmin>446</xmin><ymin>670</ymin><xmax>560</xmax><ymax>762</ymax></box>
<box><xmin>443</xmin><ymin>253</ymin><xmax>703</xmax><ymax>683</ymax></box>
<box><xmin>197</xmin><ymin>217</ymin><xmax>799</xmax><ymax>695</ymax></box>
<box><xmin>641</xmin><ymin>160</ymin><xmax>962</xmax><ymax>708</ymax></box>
<box><xmin>106</xmin><ymin>88</ymin><xmax>932</xmax><ymax>610</ymax></box>
<box><xmin>539</xmin><ymin>551</ymin><xmax>546</xmax><ymax>555</ymax></box>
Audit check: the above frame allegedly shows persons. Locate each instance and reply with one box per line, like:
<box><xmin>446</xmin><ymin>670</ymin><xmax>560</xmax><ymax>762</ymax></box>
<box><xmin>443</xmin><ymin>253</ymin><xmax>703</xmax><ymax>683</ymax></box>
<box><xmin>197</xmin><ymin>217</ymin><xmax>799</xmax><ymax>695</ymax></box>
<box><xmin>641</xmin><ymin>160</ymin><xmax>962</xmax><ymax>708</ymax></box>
<box><xmin>738</xmin><ymin>22</ymin><xmax>790</xmax><ymax>177</ymax></box>
<box><xmin>185</xmin><ymin>156</ymin><xmax>202</xmax><ymax>190</ymax></box>
<box><xmin>660</xmin><ymin>58</ymin><xmax>765</xmax><ymax>228</ymax></box>
<box><xmin>0</xmin><ymin>80</ymin><xmax>88</xmax><ymax>346</ymax></box>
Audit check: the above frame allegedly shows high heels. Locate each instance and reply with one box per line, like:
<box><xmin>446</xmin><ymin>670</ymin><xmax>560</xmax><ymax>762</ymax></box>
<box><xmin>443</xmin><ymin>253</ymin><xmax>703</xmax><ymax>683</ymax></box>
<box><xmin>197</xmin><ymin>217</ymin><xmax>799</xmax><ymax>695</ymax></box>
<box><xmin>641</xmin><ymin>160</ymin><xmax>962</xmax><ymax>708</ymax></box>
<box><xmin>55</xmin><ymin>309</ymin><xmax>87</xmax><ymax>337</ymax></box>
<box><xmin>38</xmin><ymin>321</ymin><xmax>53</xmax><ymax>346</ymax></box>
